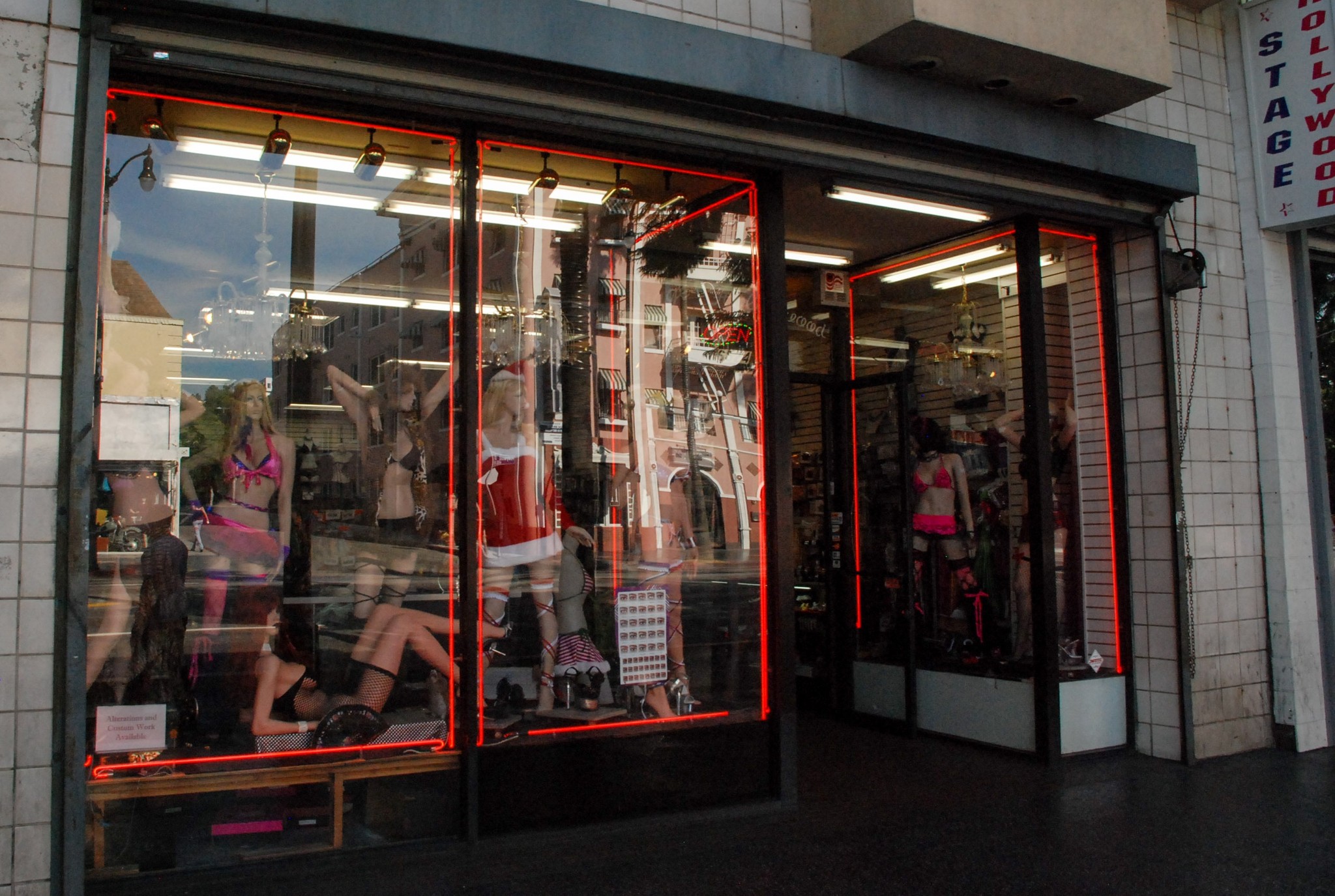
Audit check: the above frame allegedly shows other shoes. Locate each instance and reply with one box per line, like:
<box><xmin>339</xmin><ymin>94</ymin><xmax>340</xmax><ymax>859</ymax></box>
<box><xmin>496</xmin><ymin>678</ymin><xmax>510</xmax><ymax>714</ymax></box>
<box><xmin>508</xmin><ymin>684</ymin><xmax>525</xmax><ymax>715</ymax></box>
<box><xmin>485</xmin><ymin>642</ymin><xmax>497</xmax><ymax>662</ymax></box>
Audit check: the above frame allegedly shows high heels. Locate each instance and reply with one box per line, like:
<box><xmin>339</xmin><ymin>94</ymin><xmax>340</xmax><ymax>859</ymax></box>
<box><xmin>562</xmin><ymin>664</ymin><xmax>700</xmax><ymax>718</ymax></box>
<box><xmin>483</xmin><ymin>621</ymin><xmax>513</xmax><ymax>656</ymax></box>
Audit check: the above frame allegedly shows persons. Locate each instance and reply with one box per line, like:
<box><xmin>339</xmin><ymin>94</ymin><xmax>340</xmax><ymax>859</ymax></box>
<box><xmin>326</xmin><ymin>356</ymin><xmax>462</xmax><ymax>629</ymax></box>
<box><xmin>329</xmin><ymin>443</ymin><xmax>353</xmax><ymax>483</ymax></box>
<box><xmin>474</xmin><ymin>362</ymin><xmax>593</xmax><ymax>718</ymax></box>
<box><xmin>611</xmin><ymin>382</ymin><xmax>702</xmax><ymax>717</ymax></box>
<box><xmin>992</xmin><ymin>390</ymin><xmax>1082</xmax><ymax>659</ymax></box>
<box><xmin>300</xmin><ymin>435</ymin><xmax>320</xmax><ymax>482</ymax></box>
<box><xmin>228</xmin><ymin>588</ymin><xmax>503</xmax><ymax>737</ymax></box>
<box><xmin>85</xmin><ymin>348</ymin><xmax>206</xmax><ymax>704</ymax></box>
<box><xmin>181</xmin><ymin>379</ymin><xmax>294</xmax><ymax>680</ymax></box>
<box><xmin>908</xmin><ymin>416</ymin><xmax>990</xmax><ymax>642</ymax></box>
<box><xmin>556</xmin><ymin>533</ymin><xmax>612</xmax><ymax>674</ymax></box>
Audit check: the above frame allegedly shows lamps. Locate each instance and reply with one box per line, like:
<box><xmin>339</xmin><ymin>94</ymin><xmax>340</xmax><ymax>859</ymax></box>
<box><xmin>138</xmin><ymin>103</ymin><xmax>643</xmax><ymax>229</ymax></box>
<box><xmin>180</xmin><ymin>171</ymin><xmax>334</xmax><ymax>365</ymax></box>
<box><xmin>877</xmin><ymin>240</ymin><xmax>1062</xmax><ymax>295</ymax></box>
<box><xmin>781</xmin><ymin>239</ymin><xmax>854</xmax><ymax>268</ymax></box>
<box><xmin>820</xmin><ymin>179</ymin><xmax>993</xmax><ymax>228</ymax></box>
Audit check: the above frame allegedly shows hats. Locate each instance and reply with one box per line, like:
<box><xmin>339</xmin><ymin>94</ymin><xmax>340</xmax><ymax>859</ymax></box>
<box><xmin>491</xmin><ymin>359</ymin><xmax>529</xmax><ymax>409</ymax></box>
